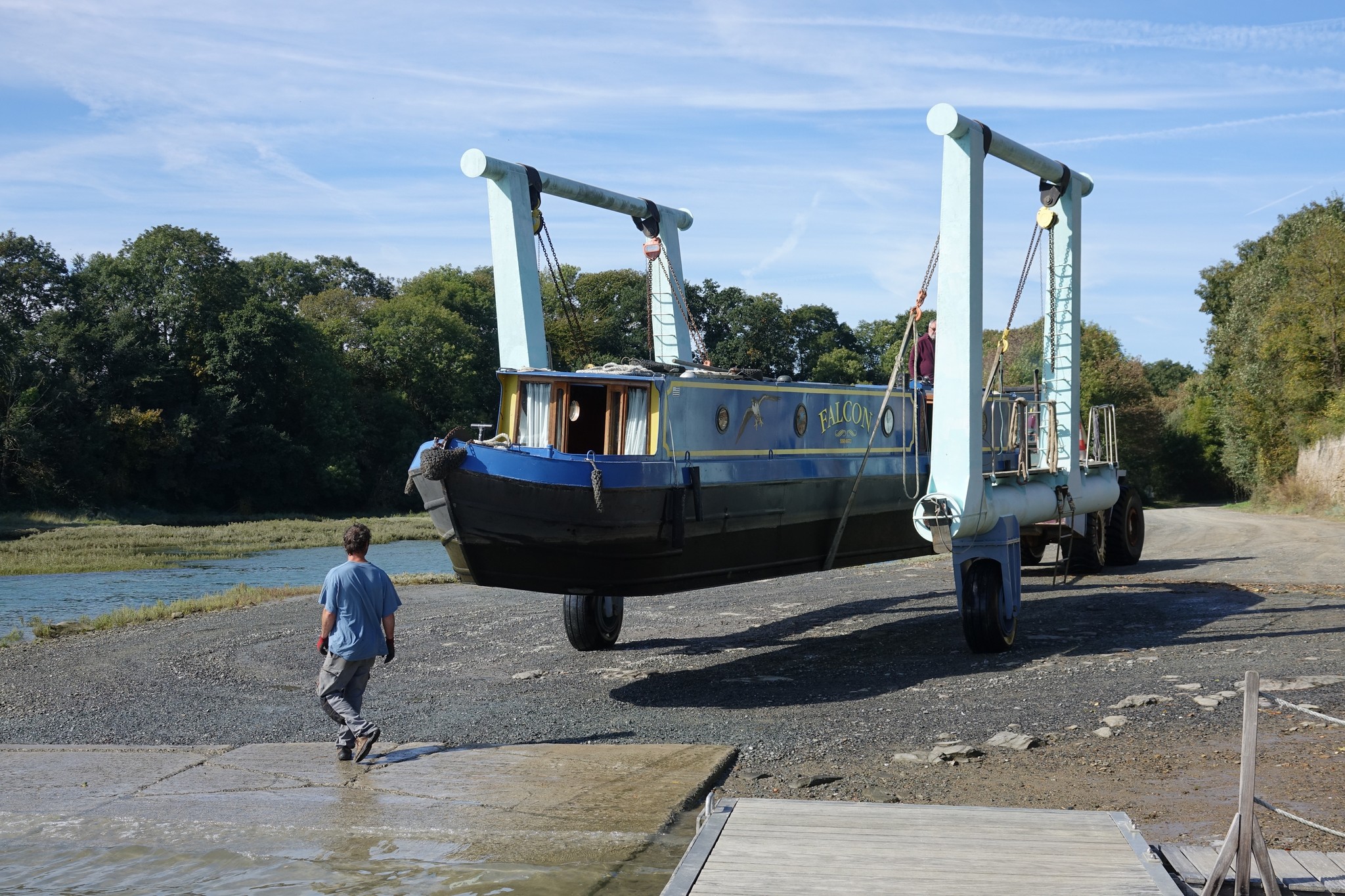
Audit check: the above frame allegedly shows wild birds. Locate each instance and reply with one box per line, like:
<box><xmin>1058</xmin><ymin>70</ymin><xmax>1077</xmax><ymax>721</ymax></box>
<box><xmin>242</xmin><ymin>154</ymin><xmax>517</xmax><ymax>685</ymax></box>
<box><xmin>735</xmin><ymin>395</ymin><xmax>781</xmax><ymax>445</ymax></box>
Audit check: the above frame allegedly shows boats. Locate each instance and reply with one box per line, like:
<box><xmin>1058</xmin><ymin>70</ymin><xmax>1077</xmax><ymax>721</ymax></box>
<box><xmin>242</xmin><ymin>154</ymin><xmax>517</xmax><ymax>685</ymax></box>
<box><xmin>400</xmin><ymin>364</ymin><xmax>1026</xmax><ymax>595</ymax></box>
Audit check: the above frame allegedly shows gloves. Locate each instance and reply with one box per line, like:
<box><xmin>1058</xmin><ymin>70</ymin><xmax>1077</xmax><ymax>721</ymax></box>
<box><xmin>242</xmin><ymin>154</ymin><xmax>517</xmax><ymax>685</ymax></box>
<box><xmin>317</xmin><ymin>633</ymin><xmax>329</xmax><ymax>655</ymax></box>
<box><xmin>382</xmin><ymin>636</ymin><xmax>395</xmax><ymax>663</ymax></box>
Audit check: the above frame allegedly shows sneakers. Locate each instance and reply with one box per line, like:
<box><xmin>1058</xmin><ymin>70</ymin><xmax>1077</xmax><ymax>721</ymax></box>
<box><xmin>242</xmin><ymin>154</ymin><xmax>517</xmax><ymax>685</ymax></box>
<box><xmin>353</xmin><ymin>729</ymin><xmax>381</xmax><ymax>762</ymax></box>
<box><xmin>337</xmin><ymin>746</ymin><xmax>352</xmax><ymax>761</ymax></box>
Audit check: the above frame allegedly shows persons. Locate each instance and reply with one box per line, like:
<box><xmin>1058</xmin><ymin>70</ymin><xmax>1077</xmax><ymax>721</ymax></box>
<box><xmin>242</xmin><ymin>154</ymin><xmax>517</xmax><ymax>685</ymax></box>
<box><xmin>316</xmin><ymin>524</ymin><xmax>403</xmax><ymax>764</ymax></box>
<box><xmin>907</xmin><ymin>318</ymin><xmax>937</xmax><ymax>390</ymax></box>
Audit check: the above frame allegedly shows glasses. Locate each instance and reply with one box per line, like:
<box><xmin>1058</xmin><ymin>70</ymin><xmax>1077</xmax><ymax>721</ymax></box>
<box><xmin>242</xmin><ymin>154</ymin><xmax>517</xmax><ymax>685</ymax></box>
<box><xmin>929</xmin><ymin>327</ymin><xmax>936</xmax><ymax>331</ymax></box>
